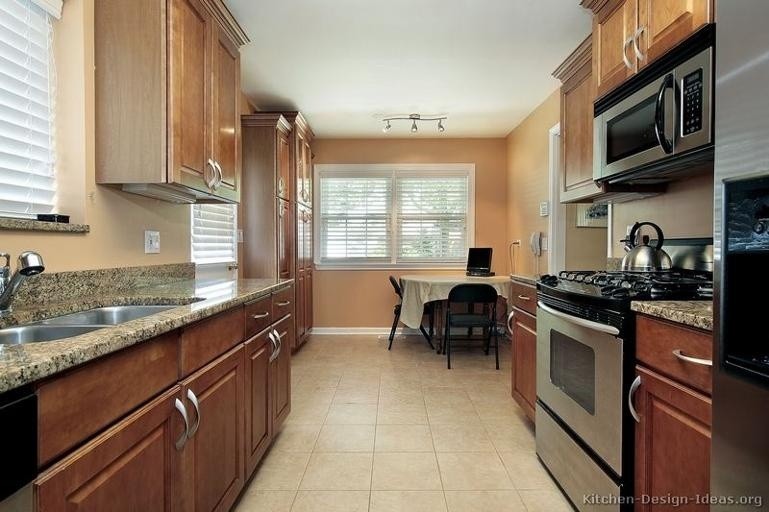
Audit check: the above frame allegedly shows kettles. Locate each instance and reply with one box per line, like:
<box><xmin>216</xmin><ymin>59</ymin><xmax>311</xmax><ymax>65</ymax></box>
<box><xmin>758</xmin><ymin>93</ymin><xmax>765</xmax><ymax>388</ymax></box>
<box><xmin>619</xmin><ymin>220</ymin><xmax>676</xmax><ymax>272</ymax></box>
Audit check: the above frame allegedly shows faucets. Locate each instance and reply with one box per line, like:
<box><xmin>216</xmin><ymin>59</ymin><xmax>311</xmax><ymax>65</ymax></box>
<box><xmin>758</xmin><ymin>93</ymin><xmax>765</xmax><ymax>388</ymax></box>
<box><xmin>0</xmin><ymin>250</ymin><xmax>47</xmax><ymax>314</ymax></box>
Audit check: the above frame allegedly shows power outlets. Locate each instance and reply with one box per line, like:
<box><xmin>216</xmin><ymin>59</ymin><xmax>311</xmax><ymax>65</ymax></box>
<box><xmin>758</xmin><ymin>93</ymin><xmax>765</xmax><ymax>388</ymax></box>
<box><xmin>513</xmin><ymin>239</ymin><xmax>521</xmax><ymax>247</ymax></box>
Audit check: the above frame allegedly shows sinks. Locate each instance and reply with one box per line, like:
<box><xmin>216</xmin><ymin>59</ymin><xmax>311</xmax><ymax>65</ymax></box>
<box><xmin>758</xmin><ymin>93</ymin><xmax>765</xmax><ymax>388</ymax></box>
<box><xmin>23</xmin><ymin>296</ymin><xmax>208</xmax><ymax>325</ymax></box>
<box><xmin>0</xmin><ymin>325</ymin><xmax>103</xmax><ymax>345</ymax></box>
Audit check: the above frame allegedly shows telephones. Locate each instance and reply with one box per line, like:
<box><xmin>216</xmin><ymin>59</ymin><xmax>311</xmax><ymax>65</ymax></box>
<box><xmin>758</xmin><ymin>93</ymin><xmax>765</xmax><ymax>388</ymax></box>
<box><xmin>529</xmin><ymin>232</ymin><xmax>541</xmax><ymax>257</ymax></box>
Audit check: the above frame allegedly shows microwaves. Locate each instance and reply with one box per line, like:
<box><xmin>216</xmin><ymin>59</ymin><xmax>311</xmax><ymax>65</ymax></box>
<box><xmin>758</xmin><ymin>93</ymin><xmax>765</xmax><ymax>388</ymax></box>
<box><xmin>581</xmin><ymin>44</ymin><xmax>713</xmax><ymax>189</ymax></box>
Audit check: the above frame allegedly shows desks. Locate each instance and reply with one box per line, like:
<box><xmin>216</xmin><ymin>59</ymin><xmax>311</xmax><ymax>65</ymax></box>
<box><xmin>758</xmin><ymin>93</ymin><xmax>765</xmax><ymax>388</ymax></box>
<box><xmin>398</xmin><ymin>275</ymin><xmax>511</xmax><ymax>356</ymax></box>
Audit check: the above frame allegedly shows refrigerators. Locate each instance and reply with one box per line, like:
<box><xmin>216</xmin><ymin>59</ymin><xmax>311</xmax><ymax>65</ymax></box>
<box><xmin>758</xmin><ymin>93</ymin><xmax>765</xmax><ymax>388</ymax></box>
<box><xmin>712</xmin><ymin>3</ymin><xmax>769</xmax><ymax>509</ymax></box>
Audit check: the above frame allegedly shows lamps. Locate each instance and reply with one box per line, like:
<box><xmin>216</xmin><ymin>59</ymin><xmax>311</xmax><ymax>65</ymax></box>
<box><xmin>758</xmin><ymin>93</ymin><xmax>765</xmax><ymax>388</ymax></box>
<box><xmin>382</xmin><ymin>113</ymin><xmax>447</xmax><ymax>133</ymax></box>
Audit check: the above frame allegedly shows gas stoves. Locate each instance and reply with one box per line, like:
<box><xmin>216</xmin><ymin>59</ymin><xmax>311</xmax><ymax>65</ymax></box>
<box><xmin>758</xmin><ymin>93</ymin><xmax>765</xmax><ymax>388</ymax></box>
<box><xmin>537</xmin><ymin>268</ymin><xmax>714</xmax><ymax>313</ymax></box>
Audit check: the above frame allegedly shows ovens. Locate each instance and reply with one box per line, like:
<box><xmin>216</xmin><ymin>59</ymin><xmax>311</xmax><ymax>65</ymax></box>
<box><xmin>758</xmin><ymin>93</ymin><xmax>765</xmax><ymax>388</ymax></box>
<box><xmin>534</xmin><ymin>305</ymin><xmax>622</xmax><ymax>511</ymax></box>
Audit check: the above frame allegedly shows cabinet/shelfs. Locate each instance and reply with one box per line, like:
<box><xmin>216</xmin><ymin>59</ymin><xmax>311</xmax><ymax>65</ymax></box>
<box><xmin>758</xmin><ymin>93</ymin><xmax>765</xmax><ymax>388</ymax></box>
<box><xmin>242</xmin><ymin>285</ymin><xmax>292</xmax><ymax>488</ymax></box>
<box><xmin>92</xmin><ymin>0</ymin><xmax>250</xmax><ymax>207</ymax></box>
<box><xmin>508</xmin><ymin>273</ymin><xmax>537</xmax><ymax>425</ymax></box>
<box><xmin>291</xmin><ymin>270</ymin><xmax>315</xmax><ymax>353</ymax></box>
<box><xmin>31</xmin><ymin>301</ymin><xmax>246</xmax><ymax>512</ymax></box>
<box><xmin>577</xmin><ymin>1</ymin><xmax>716</xmax><ymax>102</ymax></box>
<box><xmin>549</xmin><ymin>33</ymin><xmax>666</xmax><ymax>209</ymax></box>
<box><xmin>626</xmin><ymin>307</ymin><xmax>714</xmax><ymax>512</ymax></box>
<box><xmin>256</xmin><ymin>106</ymin><xmax>315</xmax><ymax>273</ymax></box>
<box><xmin>238</xmin><ymin>114</ymin><xmax>290</xmax><ymax>276</ymax></box>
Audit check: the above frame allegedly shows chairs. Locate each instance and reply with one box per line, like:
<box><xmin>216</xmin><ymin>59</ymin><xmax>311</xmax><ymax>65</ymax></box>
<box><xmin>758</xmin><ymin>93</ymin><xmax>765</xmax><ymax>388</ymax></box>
<box><xmin>386</xmin><ymin>272</ymin><xmax>435</xmax><ymax>351</ymax></box>
<box><xmin>442</xmin><ymin>283</ymin><xmax>501</xmax><ymax>370</ymax></box>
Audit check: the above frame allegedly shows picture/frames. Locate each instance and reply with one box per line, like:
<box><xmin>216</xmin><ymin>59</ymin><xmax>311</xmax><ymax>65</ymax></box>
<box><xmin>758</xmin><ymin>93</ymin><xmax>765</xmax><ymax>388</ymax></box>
<box><xmin>574</xmin><ymin>202</ymin><xmax>608</xmax><ymax>229</ymax></box>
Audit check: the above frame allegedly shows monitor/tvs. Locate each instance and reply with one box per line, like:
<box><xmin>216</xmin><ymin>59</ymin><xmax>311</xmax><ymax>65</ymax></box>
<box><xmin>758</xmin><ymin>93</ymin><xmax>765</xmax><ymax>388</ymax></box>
<box><xmin>466</xmin><ymin>248</ymin><xmax>495</xmax><ymax>276</ymax></box>
